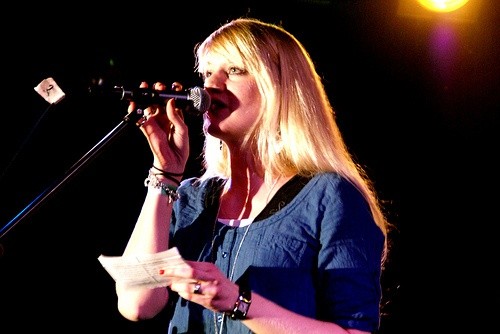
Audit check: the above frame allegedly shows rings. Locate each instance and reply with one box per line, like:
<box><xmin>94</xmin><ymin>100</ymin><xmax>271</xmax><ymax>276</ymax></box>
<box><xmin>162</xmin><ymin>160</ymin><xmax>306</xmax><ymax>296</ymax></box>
<box><xmin>136</xmin><ymin>115</ymin><xmax>148</xmax><ymax>128</ymax></box>
<box><xmin>193</xmin><ymin>284</ymin><xmax>201</xmax><ymax>293</ymax></box>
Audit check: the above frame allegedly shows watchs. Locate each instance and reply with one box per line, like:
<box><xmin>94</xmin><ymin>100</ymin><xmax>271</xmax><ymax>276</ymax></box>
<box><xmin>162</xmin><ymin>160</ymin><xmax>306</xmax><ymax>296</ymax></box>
<box><xmin>230</xmin><ymin>284</ymin><xmax>252</xmax><ymax>320</ymax></box>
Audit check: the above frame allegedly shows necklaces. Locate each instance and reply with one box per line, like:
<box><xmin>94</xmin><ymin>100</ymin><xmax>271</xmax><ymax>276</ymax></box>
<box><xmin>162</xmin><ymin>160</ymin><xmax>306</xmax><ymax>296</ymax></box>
<box><xmin>212</xmin><ymin>172</ymin><xmax>281</xmax><ymax>334</ymax></box>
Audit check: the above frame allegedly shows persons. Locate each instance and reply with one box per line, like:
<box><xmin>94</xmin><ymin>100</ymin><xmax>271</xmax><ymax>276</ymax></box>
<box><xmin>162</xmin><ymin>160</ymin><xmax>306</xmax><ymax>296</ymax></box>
<box><xmin>115</xmin><ymin>18</ymin><xmax>388</xmax><ymax>334</ymax></box>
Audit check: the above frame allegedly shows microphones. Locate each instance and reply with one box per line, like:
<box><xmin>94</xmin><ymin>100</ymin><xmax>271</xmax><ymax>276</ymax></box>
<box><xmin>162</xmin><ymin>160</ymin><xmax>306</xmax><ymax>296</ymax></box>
<box><xmin>89</xmin><ymin>80</ymin><xmax>211</xmax><ymax>117</ymax></box>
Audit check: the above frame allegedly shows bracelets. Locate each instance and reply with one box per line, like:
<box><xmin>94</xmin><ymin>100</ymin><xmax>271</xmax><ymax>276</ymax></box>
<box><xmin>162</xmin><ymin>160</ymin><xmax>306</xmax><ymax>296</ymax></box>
<box><xmin>144</xmin><ymin>170</ymin><xmax>180</xmax><ymax>203</ymax></box>
<box><xmin>151</xmin><ymin>165</ymin><xmax>183</xmax><ymax>187</ymax></box>
